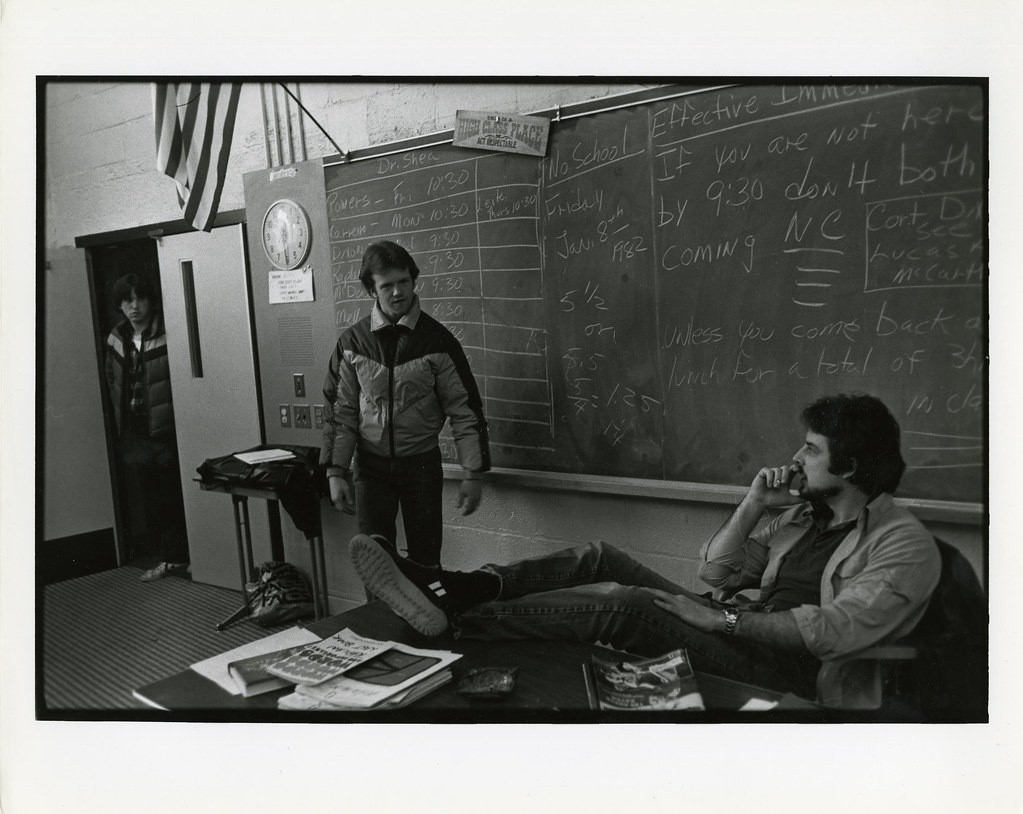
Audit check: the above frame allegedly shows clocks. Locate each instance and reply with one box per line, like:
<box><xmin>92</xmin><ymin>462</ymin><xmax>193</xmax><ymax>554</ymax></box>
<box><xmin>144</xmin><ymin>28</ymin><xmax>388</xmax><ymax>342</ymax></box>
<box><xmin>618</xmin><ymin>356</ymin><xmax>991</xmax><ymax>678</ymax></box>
<box><xmin>259</xmin><ymin>197</ymin><xmax>315</xmax><ymax>274</ymax></box>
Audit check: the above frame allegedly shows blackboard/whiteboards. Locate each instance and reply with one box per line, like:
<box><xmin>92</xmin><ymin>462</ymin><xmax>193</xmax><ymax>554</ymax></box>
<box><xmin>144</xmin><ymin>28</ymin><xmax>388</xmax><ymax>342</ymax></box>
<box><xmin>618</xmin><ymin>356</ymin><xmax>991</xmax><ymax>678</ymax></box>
<box><xmin>320</xmin><ymin>86</ymin><xmax>987</xmax><ymax>525</ymax></box>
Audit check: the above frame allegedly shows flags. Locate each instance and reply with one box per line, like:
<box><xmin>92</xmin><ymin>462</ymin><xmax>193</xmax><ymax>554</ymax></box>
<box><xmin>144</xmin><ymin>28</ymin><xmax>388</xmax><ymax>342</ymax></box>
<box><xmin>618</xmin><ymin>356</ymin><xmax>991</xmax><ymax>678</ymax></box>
<box><xmin>151</xmin><ymin>80</ymin><xmax>244</xmax><ymax>233</ymax></box>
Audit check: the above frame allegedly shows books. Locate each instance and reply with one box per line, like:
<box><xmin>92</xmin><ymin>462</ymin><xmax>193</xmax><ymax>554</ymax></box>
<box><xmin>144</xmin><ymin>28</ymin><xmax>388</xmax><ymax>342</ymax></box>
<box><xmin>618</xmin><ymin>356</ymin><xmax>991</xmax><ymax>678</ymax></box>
<box><xmin>229</xmin><ymin>627</ymin><xmax>465</xmax><ymax>715</ymax></box>
<box><xmin>583</xmin><ymin>646</ymin><xmax>706</xmax><ymax>711</ymax></box>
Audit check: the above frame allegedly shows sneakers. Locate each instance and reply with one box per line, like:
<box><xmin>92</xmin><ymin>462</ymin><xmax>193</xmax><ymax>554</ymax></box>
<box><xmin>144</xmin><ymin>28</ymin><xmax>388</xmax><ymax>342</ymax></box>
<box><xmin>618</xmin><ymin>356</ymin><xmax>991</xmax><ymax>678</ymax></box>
<box><xmin>348</xmin><ymin>534</ymin><xmax>449</xmax><ymax>638</ymax></box>
<box><xmin>141</xmin><ymin>558</ymin><xmax>189</xmax><ymax>581</ymax></box>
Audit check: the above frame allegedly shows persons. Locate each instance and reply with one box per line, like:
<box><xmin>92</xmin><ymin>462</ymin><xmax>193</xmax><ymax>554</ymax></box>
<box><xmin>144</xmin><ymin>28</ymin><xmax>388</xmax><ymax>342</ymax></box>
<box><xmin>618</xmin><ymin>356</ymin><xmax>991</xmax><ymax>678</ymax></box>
<box><xmin>318</xmin><ymin>242</ymin><xmax>489</xmax><ymax>607</ymax></box>
<box><xmin>347</xmin><ymin>393</ymin><xmax>944</xmax><ymax>711</ymax></box>
<box><xmin>102</xmin><ymin>272</ymin><xmax>188</xmax><ymax>583</ymax></box>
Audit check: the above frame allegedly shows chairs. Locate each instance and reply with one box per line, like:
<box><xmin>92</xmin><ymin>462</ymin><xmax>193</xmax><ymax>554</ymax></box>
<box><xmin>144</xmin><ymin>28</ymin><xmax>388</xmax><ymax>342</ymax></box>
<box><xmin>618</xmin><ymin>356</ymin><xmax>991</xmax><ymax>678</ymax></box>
<box><xmin>818</xmin><ymin>537</ymin><xmax>988</xmax><ymax>723</ymax></box>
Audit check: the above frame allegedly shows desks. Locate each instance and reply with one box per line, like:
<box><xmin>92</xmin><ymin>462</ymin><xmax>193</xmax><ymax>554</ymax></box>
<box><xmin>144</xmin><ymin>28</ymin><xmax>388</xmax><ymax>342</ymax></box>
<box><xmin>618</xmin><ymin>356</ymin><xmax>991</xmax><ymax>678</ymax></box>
<box><xmin>193</xmin><ymin>477</ymin><xmax>333</xmax><ymax>631</ymax></box>
<box><xmin>131</xmin><ymin>598</ymin><xmax>838</xmax><ymax>723</ymax></box>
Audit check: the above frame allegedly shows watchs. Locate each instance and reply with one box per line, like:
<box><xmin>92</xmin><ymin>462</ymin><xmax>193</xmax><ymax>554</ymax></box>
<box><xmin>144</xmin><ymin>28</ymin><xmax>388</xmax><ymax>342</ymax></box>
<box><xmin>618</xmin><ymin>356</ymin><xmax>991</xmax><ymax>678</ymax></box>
<box><xmin>722</xmin><ymin>607</ymin><xmax>741</xmax><ymax>639</ymax></box>
